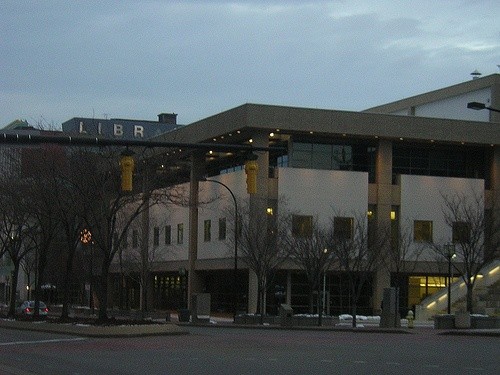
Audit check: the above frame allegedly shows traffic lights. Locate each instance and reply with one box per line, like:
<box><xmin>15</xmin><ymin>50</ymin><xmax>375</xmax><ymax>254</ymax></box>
<box><xmin>119</xmin><ymin>155</ymin><xmax>136</xmax><ymax>192</ymax></box>
<box><xmin>244</xmin><ymin>161</ymin><xmax>258</xmax><ymax>195</ymax></box>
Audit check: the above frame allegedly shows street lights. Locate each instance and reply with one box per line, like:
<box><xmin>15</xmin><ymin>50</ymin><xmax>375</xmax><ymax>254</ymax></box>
<box><xmin>317</xmin><ymin>246</ymin><xmax>327</xmax><ymax>326</ymax></box>
<box><xmin>27</xmin><ymin>285</ymin><xmax>30</xmax><ymax>300</ymax></box>
<box><xmin>197</xmin><ymin>176</ymin><xmax>239</xmax><ymax>322</ymax></box>
<box><xmin>447</xmin><ymin>253</ymin><xmax>456</xmax><ymax>313</ymax></box>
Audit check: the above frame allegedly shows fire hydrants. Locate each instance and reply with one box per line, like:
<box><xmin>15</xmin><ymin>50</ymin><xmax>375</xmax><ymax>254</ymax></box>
<box><xmin>405</xmin><ymin>309</ymin><xmax>414</xmax><ymax>329</ymax></box>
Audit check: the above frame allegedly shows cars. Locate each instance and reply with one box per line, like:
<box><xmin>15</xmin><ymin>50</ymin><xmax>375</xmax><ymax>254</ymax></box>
<box><xmin>15</xmin><ymin>300</ymin><xmax>48</xmax><ymax>320</ymax></box>
